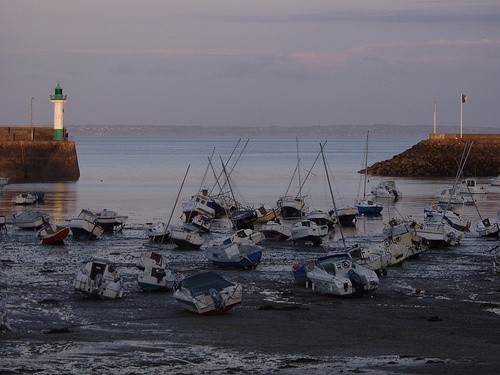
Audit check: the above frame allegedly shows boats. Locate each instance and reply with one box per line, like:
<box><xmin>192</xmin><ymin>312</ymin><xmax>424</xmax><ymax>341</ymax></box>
<box><xmin>64</xmin><ymin>208</ymin><xmax>129</xmax><ymax>239</ymax></box>
<box><xmin>263</xmin><ymin>291</ymin><xmax>311</xmax><ymax>310</ymax></box>
<box><xmin>12</xmin><ymin>210</ymin><xmax>50</xmax><ymax>229</ymax></box>
<box><xmin>0</xmin><ymin>216</ymin><xmax>6</xmax><ymax>226</ymax></box>
<box><xmin>0</xmin><ymin>174</ymin><xmax>10</xmax><ymax>185</ymax></box>
<box><xmin>73</xmin><ymin>256</ymin><xmax>123</xmax><ymax>298</ymax></box>
<box><xmin>37</xmin><ymin>223</ymin><xmax>70</xmax><ymax>243</ymax></box>
<box><xmin>172</xmin><ymin>270</ymin><xmax>242</xmax><ymax>317</ymax></box>
<box><xmin>10</xmin><ymin>192</ymin><xmax>36</xmax><ymax>204</ymax></box>
<box><xmin>304</xmin><ymin>251</ymin><xmax>380</xmax><ymax>297</ymax></box>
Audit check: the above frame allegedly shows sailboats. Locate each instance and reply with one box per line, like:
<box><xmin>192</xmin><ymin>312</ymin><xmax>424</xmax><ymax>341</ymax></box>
<box><xmin>135</xmin><ymin>129</ymin><xmax>500</xmax><ymax>294</ymax></box>
